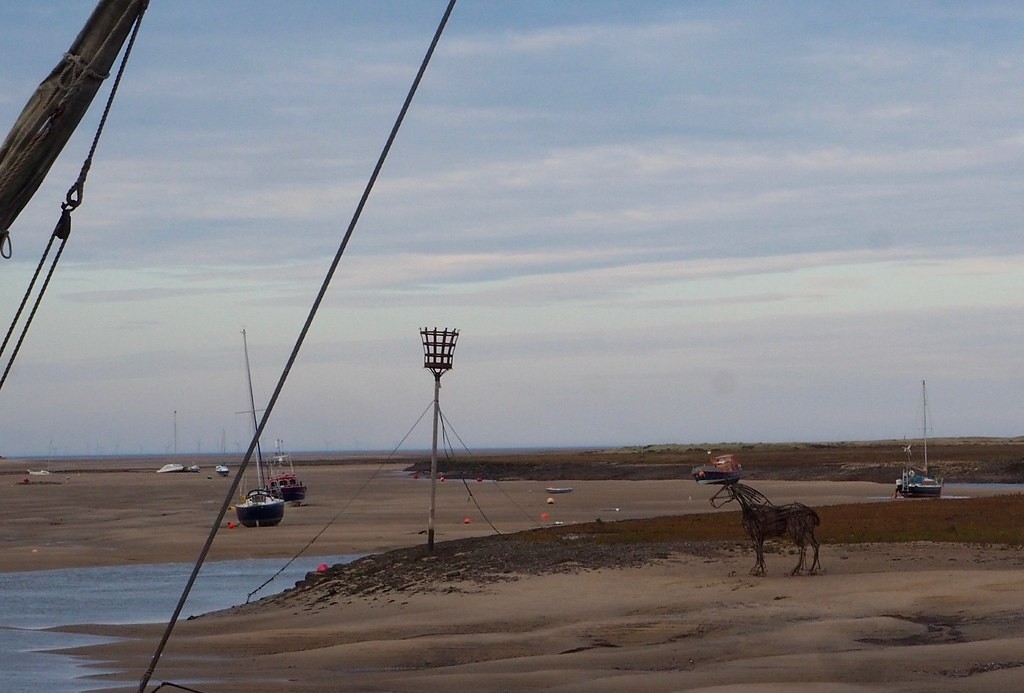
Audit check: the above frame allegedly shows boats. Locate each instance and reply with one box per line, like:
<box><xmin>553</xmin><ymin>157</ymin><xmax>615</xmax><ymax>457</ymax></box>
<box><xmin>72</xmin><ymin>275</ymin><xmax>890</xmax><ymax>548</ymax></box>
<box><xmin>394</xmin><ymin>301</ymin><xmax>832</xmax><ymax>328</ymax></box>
<box><xmin>215</xmin><ymin>465</ymin><xmax>229</xmax><ymax>476</ymax></box>
<box><xmin>690</xmin><ymin>450</ymin><xmax>743</xmax><ymax>484</ymax></box>
<box><xmin>27</xmin><ymin>440</ymin><xmax>56</xmax><ymax>475</ymax></box>
<box><xmin>263</xmin><ymin>436</ymin><xmax>307</xmax><ymax>502</ymax></box>
<box><xmin>155</xmin><ymin>463</ymin><xmax>184</xmax><ymax>473</ymax></box>
<box><xmin>545</xmin><ymin>487</ymin><xmax>573</xmax><ymax>494</ymax></box>
<box><xmin>182</xmin><ymin>466</ymin><xmax>200</xmax><ymax>472</ymax></box>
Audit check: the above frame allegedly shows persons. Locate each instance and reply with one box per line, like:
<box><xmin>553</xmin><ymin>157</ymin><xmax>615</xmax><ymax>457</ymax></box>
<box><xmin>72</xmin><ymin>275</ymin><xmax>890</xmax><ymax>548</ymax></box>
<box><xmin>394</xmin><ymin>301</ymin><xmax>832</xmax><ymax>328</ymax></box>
<box><xmin>894</xmin><ymin>477</ymin><xmax>902</xmax><ymax>499</ymax></box>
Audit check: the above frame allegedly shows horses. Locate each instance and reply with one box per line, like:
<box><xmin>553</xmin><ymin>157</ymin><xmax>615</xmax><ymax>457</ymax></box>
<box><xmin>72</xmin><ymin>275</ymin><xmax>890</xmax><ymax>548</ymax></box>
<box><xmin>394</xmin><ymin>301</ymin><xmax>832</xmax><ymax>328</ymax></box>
<box><xmin>710</xmin><ymin>478</ymin><xmax>821</xmax><ymax>577</ymax></box>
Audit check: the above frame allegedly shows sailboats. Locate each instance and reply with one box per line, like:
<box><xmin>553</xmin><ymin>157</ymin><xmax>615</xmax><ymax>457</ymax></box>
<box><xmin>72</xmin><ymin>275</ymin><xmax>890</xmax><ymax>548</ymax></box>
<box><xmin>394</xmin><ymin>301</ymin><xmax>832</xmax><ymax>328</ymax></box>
<box><xmin>234</xmin><ymin>328</ymin><xmax>286</xmax><ymax>526</ymax></box>
<box><xmin>894</xmin><ymin>379</ymin><xmax>946</xmax><ymax>499</ymax></box>
